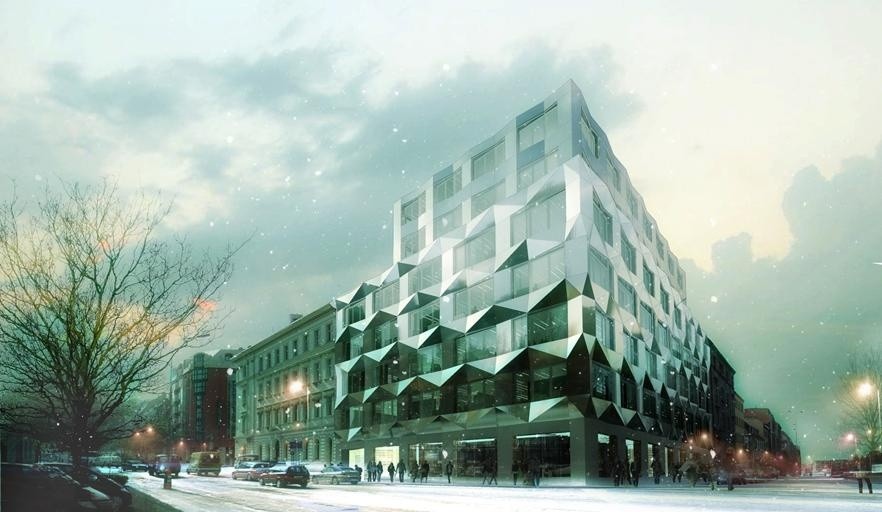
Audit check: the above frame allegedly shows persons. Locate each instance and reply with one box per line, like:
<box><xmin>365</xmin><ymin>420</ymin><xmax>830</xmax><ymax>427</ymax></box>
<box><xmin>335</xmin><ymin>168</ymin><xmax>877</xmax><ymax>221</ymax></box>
<box><xmin>367</xmin><ymin>460</ymin><xmax>372</xmax><ymax>482</ymax></box>
<box><xmin>480</xmin><ymin>456</ymin><xmax>683</xmax><ymax>488</ymax></box>
<box><xmin>387</xmin><ymin>463</ymin><xmax>394</xmax><ymax>484</ymax></box>
<box><xmin>354</xmin><ymin>464</ymin><xmax>362</xmax><ymax>482</ymax></box>
<box><xmin>445</xmin><ymin>460</ymin><xmax>453</xmax><ymax>484</ymax></box>
<box><xmin>409</xmin><ymin>460</ymin><xmax>418</xmax><ymax>483</ymax></box>
<box><xmin>376</xmin><ymin>461</ymin><xmax>382</xmax><ymax>482</ymax></box>
<box><xmin>419</xmin><ymin>459</ymin><xmax>428</xmax><ymax>484</ymax></box>
<box><xmin>372</xmin><ymin>462</ymin><xmax>376</xmax><ymax>481</ymax></box>
<box><xmin>855</xmin><ymin>449</ymin><xmax>874</xmax><ymax>494</ymax></box>
<box><xmin>395</xmin><ymin>459</ymin><xmax>405</xmax><ymax>483</ymax></box>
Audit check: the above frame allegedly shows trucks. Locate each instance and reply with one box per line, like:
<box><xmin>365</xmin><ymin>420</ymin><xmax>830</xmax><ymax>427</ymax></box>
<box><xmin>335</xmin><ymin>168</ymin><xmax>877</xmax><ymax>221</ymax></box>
<box><xmin>146</xmin><ymin>452</ymin><xmax>182</xmax><ymax>477</ymax></box>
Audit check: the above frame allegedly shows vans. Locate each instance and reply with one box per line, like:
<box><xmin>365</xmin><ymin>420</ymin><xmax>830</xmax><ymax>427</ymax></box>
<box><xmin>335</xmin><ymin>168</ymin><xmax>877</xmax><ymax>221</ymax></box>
<box><xmin>185</xmin><ymin>449</ymin><xmax>223</xmax><ymax>478</ymax></box>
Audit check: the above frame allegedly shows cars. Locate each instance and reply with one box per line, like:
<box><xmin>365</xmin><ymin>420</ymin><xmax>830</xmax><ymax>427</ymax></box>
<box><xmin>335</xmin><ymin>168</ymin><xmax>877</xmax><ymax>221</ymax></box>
<box><xmin>312</xmin><ymin>463</ymin><xmax>363</xmax><ymax>488</ymax></box>
<box><xmin>231</xmin><ymin>457</ymin><xmax>312</xmax><ymax>489</ymax></box>
<box><xmin>2</xmin><ymin>460</ymin><xmax>135</xmax><ymax>512</ymax></box>
<box><xmin>80</xmin><ymin>447</ymin><xmax>148</xmax><ymax>473</ymax></box>
<box><xmin>710</xmin><ymin>461</ymin><xmax>782</xmax><ymax>485</ymax></box>
